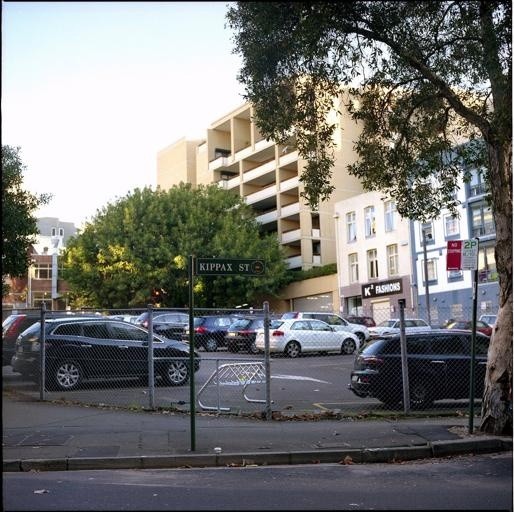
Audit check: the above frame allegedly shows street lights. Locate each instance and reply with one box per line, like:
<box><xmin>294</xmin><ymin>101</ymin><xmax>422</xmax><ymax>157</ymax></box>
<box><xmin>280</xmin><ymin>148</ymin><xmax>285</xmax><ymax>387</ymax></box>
<box><xmin>51</xmin><ymin>237</ymin><xmax>60</xmax><ymax>309</ymax></box>
<box><xmin>26</xmin><ymin>258</ymin><xmax>36</xmax><ymax>309</ymax></box>
